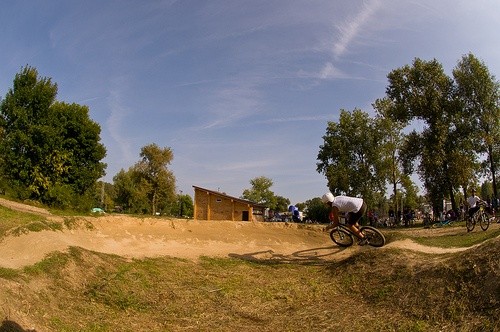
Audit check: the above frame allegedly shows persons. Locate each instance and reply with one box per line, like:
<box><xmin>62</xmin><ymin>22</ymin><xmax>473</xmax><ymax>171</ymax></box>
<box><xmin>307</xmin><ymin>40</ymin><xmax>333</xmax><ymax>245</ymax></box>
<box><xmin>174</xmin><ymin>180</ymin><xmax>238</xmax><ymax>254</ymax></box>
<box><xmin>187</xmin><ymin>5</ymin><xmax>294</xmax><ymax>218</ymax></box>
<box><xmin>321</xmin><ymin>192</ymin><xmax>368</xmax><ymax>246</ymax></box>
<box><xmin>366</xmin><ymin>188</ymin><xmax>500</xmax><ymax>226</ymax></box>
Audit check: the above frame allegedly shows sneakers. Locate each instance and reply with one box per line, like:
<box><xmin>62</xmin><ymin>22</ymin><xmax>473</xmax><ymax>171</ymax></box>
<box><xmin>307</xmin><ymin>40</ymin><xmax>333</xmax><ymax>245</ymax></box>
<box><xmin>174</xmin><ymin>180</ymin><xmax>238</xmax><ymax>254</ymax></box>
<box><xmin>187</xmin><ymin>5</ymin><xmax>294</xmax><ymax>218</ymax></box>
<box><xmin>357</xmin><ymin>239</ymin><xmax>369</xmax><ymax>245</ymax></box>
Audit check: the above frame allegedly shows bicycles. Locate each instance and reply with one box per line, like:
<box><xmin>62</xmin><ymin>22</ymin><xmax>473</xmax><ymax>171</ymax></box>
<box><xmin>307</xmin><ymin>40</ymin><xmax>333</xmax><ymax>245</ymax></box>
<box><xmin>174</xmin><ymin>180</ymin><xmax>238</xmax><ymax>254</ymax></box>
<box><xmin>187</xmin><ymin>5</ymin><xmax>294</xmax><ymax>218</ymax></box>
<box><xmin>323</xmin><ymin>221</ymin><xmax>385</xmax><ymax>247</ymax></box>
<box><xmin>465</xmin><ymin>201</ymin><xmax>492</xmax><ymax>231</ymax></box>
<box><xmin>432</xmin><ymin>219</ymin><xmax>456</xmax><ymax>229</ymax></box>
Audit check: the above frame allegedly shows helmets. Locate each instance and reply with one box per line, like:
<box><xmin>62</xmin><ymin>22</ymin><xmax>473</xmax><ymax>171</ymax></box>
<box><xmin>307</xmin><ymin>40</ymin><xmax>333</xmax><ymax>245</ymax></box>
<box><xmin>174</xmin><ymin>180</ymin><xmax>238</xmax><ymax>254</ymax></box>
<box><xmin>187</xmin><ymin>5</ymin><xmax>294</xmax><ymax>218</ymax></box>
<box><xmin>322</xmin><ymin>192</ymin><xmax>334</xmax><ymax>204</ymax></box>
